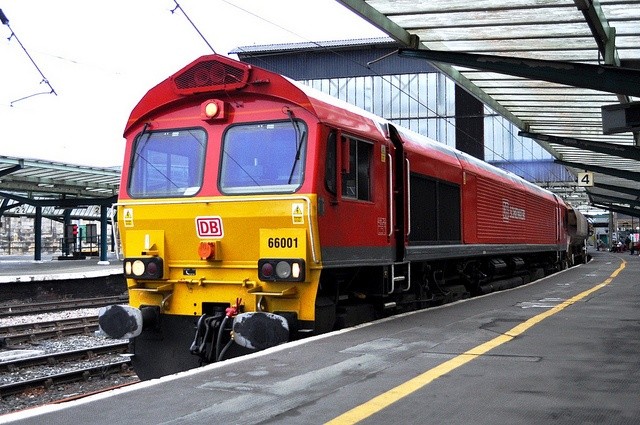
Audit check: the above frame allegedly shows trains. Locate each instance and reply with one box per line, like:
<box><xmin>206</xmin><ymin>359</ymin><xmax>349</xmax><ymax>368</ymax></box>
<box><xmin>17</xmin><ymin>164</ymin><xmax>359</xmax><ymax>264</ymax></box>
<box><xmin>98</xmin><ymin>53</ymin><xmax>589</xmax><ymax>381</ymax></box>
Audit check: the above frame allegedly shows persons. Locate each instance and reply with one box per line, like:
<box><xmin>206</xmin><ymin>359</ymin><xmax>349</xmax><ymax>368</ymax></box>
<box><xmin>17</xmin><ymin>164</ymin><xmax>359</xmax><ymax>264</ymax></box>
<box><xmin>611</xmin><ymin>236</ymin><xmax>640</xmax><ymax>256</ymax></box>
<box><xmin>597</xmin><ymin>239</ymin><xmax>605</xmax><ymax>252</ymax></box>
